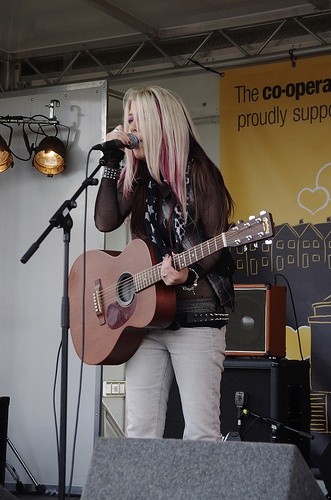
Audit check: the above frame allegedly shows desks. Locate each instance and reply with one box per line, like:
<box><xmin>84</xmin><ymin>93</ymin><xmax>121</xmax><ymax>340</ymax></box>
<box><xmin>163</xmin><ymin>358</ymin><xmax>287</xmax><ymax>441</ymax></box>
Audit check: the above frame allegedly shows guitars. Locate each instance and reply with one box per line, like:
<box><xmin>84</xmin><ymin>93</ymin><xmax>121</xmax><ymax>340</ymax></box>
<box><xmin>68</xmin><ymin>209</ymin><xmax>276</xmax><ymax>365</ymax></box>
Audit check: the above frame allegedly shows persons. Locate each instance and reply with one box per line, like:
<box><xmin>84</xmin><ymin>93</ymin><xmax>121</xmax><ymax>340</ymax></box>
<box><xmin>94</xmin><ymin>86</ymin><xmax>235</xmax><ymax>441</ymax></box>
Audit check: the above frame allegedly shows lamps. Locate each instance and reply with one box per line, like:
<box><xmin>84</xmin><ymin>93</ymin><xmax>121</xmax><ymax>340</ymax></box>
<box><xmin>0</xmin><ymin>134</ymin><xmax>14</xmax><ymax>174</ymax></box>
<box><xmin>32</xmin><ymin>136</ymin><xmax>66</xmax><ymax>178</ymax></box>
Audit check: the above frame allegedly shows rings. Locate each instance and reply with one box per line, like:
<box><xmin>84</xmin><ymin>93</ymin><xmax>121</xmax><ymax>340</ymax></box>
<box><xmin>114</xmin><ymin>129</ymin><xmax>118</xmax><ymax>131</ymax></box>
<box><xmin>163</xmin><ymin>274</ymin><xmax>167</xmax><ymax>278</ymax></box>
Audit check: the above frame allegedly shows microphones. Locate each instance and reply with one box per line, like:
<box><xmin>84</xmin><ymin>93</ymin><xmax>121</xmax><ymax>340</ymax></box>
<box><xmin>235</xmin><ymin>391</ymin><xmax>248</xmax><ymax>429</ymax></box>
<box><xmin>91</xmin><ymin>134</ymin><xmax>139</xmax><ymax>151</ymax></box>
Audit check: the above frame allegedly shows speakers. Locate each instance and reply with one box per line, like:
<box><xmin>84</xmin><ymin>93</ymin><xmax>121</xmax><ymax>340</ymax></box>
<box><xmin>80</xmin><ymin>283</ymin><xmax>326</xmax><ymax>500</ymax></box>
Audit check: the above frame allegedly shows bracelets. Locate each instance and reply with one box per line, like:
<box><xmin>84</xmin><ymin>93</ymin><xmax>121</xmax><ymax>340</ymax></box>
<box><xmin>181</xmin><ymin>268</ymin><xmax>199</xmax><ymax>291</ymax></box>
<box><xmin>104</xmin><ymin>168</ymin><xmax>121</xmax><ymax>180</ymax></box>
